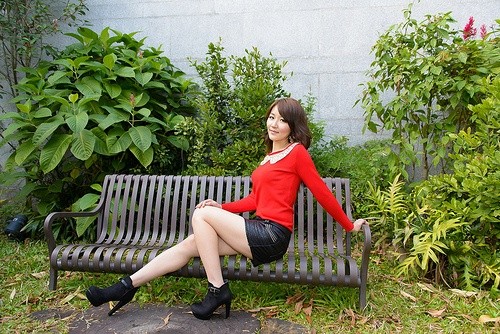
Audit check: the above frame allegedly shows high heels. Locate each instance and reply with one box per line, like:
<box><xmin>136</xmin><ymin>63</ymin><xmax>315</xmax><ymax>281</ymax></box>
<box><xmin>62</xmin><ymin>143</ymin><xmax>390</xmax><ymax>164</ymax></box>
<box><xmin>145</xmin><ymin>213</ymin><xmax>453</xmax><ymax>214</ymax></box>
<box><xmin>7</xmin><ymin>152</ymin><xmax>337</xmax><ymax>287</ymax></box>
<box><xmin>191</xmin><ymin>280</ymin><xmax>232</xmax><ymax>320</ymax></box>
<box><xmin>86</xmin><ymin>276</ymin><xmax>141</xmax><ymax>316</ymax></box>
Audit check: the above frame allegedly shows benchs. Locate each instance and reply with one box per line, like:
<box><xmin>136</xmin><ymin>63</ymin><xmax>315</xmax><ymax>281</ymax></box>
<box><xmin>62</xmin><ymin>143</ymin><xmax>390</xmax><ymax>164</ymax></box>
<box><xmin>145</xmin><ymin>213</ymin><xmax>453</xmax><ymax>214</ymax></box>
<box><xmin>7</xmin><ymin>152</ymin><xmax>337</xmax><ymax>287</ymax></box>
<box><xmin>43</xmin><ymin>172</ymin><xmax>372</xmax><ymax>313</ymax></box>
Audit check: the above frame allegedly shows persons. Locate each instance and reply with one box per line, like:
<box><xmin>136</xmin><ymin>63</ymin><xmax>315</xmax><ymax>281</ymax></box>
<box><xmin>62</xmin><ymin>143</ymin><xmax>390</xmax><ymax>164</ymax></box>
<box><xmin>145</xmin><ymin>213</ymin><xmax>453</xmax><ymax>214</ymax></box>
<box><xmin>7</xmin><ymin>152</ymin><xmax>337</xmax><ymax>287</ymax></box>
<box><xmin>84</xmin><ymin>98</ymin><xmax>371</xmax><ymax>320</ymax></box>
